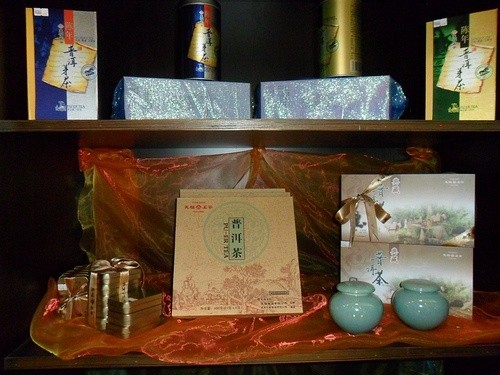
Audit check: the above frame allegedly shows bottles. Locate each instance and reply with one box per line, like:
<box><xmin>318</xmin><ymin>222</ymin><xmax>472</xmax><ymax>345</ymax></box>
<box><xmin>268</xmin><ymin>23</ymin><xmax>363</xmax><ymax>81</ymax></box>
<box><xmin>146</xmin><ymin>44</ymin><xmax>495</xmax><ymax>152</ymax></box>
<box><xmin>394</xmin><ymin>279</ymin><xmax>451</xmax><ymax>330</ymax></box>
<box><xmin>330</xmin><ymin>281</ymin><xmax>383</xmax><ymax>333</ymax></box>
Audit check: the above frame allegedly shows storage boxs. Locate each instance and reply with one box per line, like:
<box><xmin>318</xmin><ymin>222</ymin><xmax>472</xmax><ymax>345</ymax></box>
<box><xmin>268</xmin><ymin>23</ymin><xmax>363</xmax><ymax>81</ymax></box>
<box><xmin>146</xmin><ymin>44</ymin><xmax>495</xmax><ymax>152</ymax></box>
<box><xmin>26</xmin><ymin>8</ymin><xmax>99</xmax><ymax>119</ymax></box>
<box><xmin>112</xmin><ymin>76</ymin><xmax>250</xmax><ymax>120</ymax></box>
<box><xmin>425</xmin><ymin>8</ymin><xmax>500</xmax><ymax>119</ymax></box>
<box><xmin>255</xmin><ymin>74</ymin><xmax>408</xmax><ymax>120</ymax></box>
<box><xmin>340</xmin><ymin>173</ymin><xmax>474</xmax><ymax>319</ymax></box>
<box><xmin>56</xmin><ymin>265</ymin><xmax>164</xmax><ymax>338</ymax></box>
<box><xmin>172</xmin><ymin>188</ymin><xmax>304</xmax><ymax>316</ymax></box>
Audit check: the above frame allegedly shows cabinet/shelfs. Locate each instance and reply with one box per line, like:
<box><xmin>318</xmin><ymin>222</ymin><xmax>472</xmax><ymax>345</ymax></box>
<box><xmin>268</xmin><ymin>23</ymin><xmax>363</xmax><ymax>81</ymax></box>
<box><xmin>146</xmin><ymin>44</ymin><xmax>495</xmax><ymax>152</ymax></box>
<box><xmin>0</xmin><ymin>120</ymin><xmax>500</xmax><ymax>370</ymax></box>
